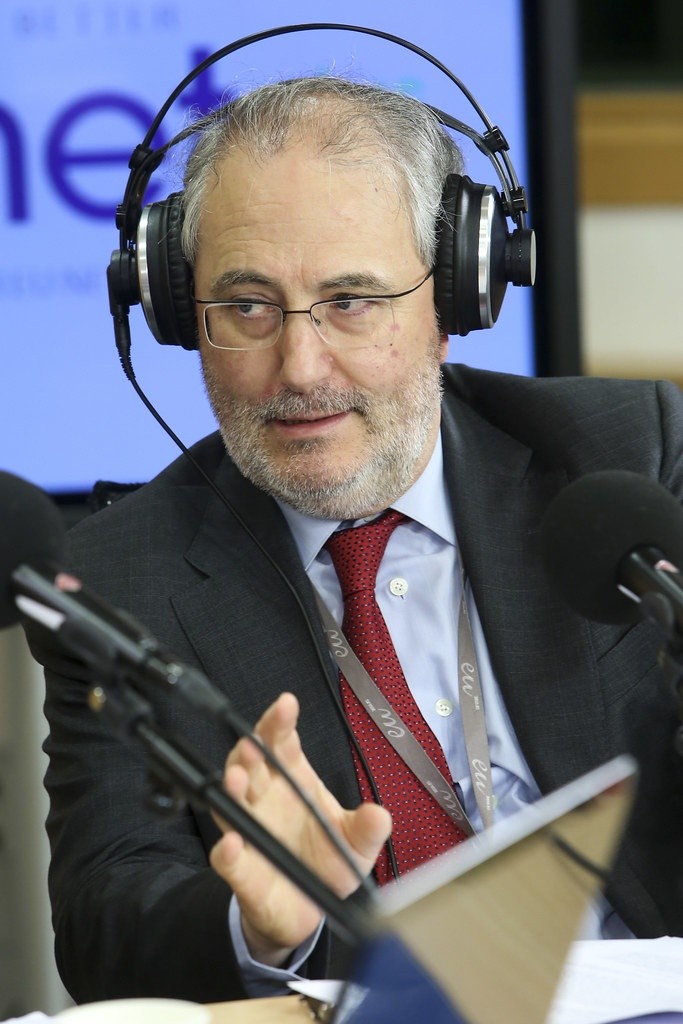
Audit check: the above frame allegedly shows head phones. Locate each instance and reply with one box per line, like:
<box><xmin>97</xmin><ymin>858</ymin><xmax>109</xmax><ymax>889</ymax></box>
<box><xmin>105</xmin><ymin>22</ymin><xmax>534</xmax><ymax>382</ymax></box>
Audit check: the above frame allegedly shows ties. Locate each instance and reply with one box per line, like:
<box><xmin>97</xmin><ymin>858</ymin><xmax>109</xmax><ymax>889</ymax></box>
<box><xmin>321</xmin><ymin>511</ymin><xmax>470</xmax><ymax>887</ymax></box>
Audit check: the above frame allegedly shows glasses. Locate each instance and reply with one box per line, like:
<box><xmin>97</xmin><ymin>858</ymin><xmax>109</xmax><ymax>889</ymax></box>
<box><xmin>187</xmin><ymin>267</ymin><xmax>438</xmax><ymax>351</ymax></box>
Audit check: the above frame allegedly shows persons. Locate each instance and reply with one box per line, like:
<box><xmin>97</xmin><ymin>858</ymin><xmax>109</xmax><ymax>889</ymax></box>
<box><xmin>26</xmin><ymin>70</ymin><xmax>683</xmax><ymax>1003</ymax></box>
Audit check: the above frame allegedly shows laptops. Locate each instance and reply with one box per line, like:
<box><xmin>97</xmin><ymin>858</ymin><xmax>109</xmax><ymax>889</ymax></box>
<box><xmin>370</xmin><ymin>756</ymin><xmax>645</xmax><ymax>1023</ymax></box>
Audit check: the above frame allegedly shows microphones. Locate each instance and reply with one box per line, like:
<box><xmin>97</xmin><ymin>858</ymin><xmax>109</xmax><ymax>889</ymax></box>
<box><xmin>535</xmin><ymin>468</ymin><xmax>682</xmax><ymax>650</ymax></box>
<box><xmin>0</xmin><ymin>467</ymin><xmax>232</xmax><ymax>728</ymax></box>
